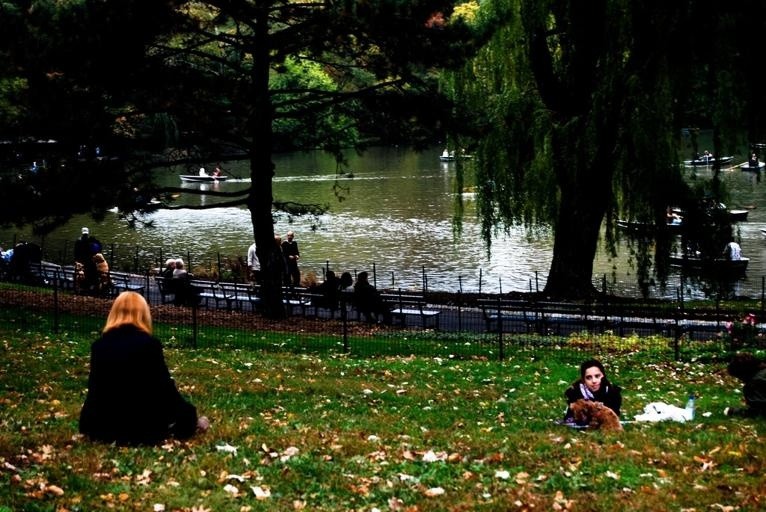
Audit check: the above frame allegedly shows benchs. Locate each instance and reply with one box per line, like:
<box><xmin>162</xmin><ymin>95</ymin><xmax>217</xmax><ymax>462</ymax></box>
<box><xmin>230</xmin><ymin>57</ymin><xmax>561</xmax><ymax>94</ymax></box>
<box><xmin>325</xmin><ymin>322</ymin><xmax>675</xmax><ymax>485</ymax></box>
<box><xmin>155</xmin><ymin>275</ymin><xmax>442</xmax><ymax>329</ymax></box>
<box><xmin>476</xmin><ymin>297</ymin><xmax>692</xmax><ymax>341</ymax></box>
<box><xmin>30</xmin><ymin>262</ymin><xmax>145</xmax><ymax>298</ymax></box>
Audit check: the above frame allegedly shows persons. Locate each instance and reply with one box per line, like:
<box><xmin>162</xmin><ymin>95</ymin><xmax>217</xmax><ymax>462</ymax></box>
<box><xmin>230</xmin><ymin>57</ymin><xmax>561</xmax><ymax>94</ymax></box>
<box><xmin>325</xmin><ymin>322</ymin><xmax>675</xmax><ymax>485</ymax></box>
<box><xmin>213</xmin><ymin>165</ymin><xmax>224</xmax><ymax>176</ymax></box>
<box><xmin>304</xmin><ymin>271</ymin><xmax>352</xmax><ymax>306</ymax></box>
<box><xmin>723</xmin><ymin>353</ymin><xmax>766</xmax><ymax>418</ymax></box>
<box><xmin>704</xmin><ymin>150</ymin><xmax>713</xmax><ymax>158</ymax></box>
<box><xmin>247</xmin><ymin>231</ymin><xmax>301</xmax><ymax>286</ymax></box>
<box><xmin>353</xmin><ymin>272</ymin><xmax>392</xmax><ymax>325</ymax></box>
<box><xmin>563</xmin><ymin>359</ymin><xmax>622</xmax><ymax>422</ymax></box>
<box><xmin>722</xmin><ymin>237</ymin><xmax>741</xmax><ymax>260</ymax></box>
<box><xmin>442</xmin><ymin>148</ymin><xmax>457</xmax><ymax>157</ymax></box>
<box><xmin>73</xmin><ymin>227</ymin><xmax>103</xmax><ymax>294</ymax></box>
<box><xmin>0</xmin><ymin>240</ymin><xmax>42</xmax><ymax>284</ymax></box>
<box><xmin>79</xmin><ymin>291</ymin><xmax>210</xmax><ymax>447</ymax></box>
<box><xmin>162</xmin><ymin>259</ymin><xmax>204</xmax><ymax>305</ymax></box>
<box><xmin>199</xmin><ymin>167</ymin><xmax>210</xmax><ymax>176</ymax></box>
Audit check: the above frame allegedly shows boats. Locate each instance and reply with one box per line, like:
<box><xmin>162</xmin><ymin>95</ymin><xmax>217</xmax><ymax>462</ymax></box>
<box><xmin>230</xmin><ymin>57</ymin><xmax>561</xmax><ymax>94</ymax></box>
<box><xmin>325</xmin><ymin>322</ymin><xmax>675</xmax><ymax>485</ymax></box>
<box><xmin>612</xmin><ymin>210</ymin><xmax>749</xmax><ymax>234</ymax></box>
<box><xmin>666</xmin><ymin>250</ymin><xmax>750</xmax><ymax>275</ymax></box>
<box><xmin>739</xmin><ymin>160</ymin><xmax>765</xmax><ymax>170</ymax></box>
<box><xmin>681</xmin><ymin>156</ymin><xmax>734</xmax><ymax>167</ymax></box>
<box><xmin>440</xmin><ymin>155</ymin><xmax>474</xmax><ymax>161</ymax></box>
<box><xmin>178</xmin><ymin>174</ymin><xmax>228</xmax><ymax>183</ymax></box>
